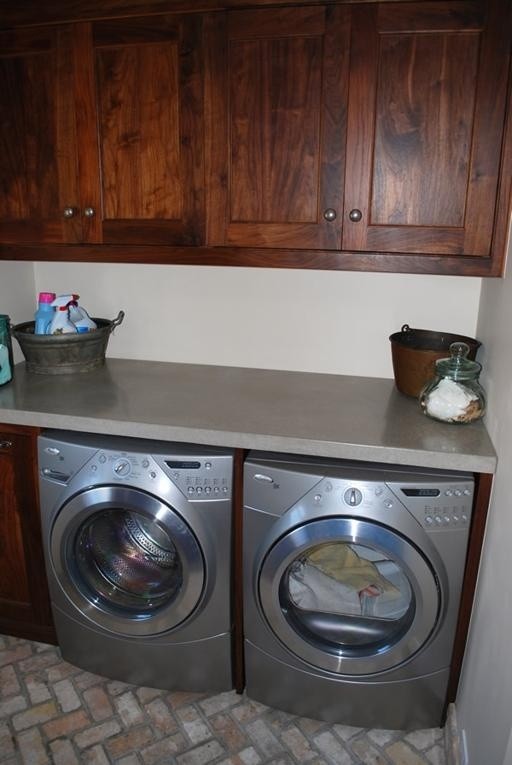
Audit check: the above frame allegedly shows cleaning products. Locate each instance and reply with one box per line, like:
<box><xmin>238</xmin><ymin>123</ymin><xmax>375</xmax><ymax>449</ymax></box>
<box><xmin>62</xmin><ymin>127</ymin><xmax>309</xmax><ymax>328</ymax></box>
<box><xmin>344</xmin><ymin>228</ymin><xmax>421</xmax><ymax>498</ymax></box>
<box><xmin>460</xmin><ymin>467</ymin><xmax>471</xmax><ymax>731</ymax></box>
<box><xmin>35</xmin><ymin>292</ymin><xmax>98</xmax><ymax>335</ymax></box>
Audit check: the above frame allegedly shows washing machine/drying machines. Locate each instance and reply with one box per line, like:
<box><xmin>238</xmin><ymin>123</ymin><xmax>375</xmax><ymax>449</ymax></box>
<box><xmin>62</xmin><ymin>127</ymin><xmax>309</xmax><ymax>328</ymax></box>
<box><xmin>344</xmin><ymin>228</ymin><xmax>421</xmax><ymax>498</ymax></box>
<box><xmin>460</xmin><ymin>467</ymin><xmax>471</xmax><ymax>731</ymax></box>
<box><xmin>241</xmin><ymin>448</ymin><xmax>475</xmax><ymax>732</ymax></box>
<box><xmin>37</xmin><ymin>433</ymin><xmax>234</xmax><ymax>693</ymax></box>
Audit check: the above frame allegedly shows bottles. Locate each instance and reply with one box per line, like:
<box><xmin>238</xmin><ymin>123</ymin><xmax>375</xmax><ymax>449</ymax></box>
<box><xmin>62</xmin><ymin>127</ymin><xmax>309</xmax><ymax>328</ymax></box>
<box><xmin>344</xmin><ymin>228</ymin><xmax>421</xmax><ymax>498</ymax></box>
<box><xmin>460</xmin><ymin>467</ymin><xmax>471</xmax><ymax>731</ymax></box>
<box><xmin>420</xmin><ymin>338</ymin><xmax>487</xmax><ymax>426</ymax></box>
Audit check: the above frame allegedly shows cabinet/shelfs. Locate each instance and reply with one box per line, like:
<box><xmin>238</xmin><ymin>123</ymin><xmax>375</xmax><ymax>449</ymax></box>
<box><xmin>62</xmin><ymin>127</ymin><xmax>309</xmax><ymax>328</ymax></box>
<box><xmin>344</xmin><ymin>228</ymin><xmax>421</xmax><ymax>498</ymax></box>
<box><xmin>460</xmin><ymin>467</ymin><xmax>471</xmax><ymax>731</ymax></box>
<box><xmin>206</xmin><ymin>0</ymin><xmax>512</xmax><ymax>279</ymax></box>
<box><xmin>0</xmin><ymin>3</ymin><xmax>205</xmax><ymax>266</ymax></box>
<box><xmin>1</xmin><ymin>422</ymin><xmax>59</xmax><ymax>647</ymax></box>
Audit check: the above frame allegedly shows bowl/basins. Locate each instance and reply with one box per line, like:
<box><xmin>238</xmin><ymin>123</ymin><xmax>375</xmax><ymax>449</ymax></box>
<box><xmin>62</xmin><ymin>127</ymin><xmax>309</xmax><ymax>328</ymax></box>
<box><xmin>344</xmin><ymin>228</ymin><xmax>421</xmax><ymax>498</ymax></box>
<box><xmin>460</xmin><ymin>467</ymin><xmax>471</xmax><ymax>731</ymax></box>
<box><xmin>390</xmin><ymin>327</ymin><xmax>483</xmax><ymax>401</ymax></box>
<box><xmin>11</xmin><ymin>311</ymin><xmax>125</xmax><ymax>373</ymax></box>
